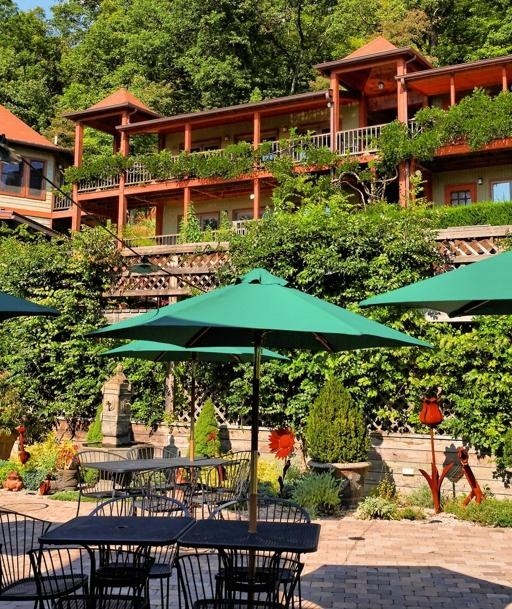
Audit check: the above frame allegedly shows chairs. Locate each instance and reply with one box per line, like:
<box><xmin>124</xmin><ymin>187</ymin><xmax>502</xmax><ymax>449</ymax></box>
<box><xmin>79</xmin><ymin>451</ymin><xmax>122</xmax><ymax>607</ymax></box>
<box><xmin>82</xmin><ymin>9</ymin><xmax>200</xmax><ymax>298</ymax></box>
<box><xmin>0</xmin><ymin>442</ymin><xmax>323</xmax><ymax>609</ymax></box>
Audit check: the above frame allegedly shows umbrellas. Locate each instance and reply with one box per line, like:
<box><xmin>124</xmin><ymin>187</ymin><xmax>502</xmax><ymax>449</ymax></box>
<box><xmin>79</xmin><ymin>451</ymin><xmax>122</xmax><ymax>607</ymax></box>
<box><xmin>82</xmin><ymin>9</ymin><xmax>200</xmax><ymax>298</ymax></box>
<box><xmin>84</xmin><ymin>267</ymin><xmax>434</xmax><ymax>609</ymax></box>
<box><xmin>357</xmin><ymin>250</ymin><xmax>512</xmax><ymax>319</ymax></box>
<box><xmin>96</xmin><ymin>339</ymin><xmax>292</xmax><ymax>488</ymax></box>
<box><xmin>0</xmin><ymin>293</ymin><xmax>61</xmax><ymax>324</ymax></box>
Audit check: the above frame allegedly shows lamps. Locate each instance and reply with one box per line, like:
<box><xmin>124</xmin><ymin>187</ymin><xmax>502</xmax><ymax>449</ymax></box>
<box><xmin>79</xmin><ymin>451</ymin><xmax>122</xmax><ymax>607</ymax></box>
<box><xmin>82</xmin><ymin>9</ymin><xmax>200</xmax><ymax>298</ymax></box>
<box><xmin>477</xmin><ymin>173</ymin><xmax>482</xmax><ymax>185</ymax></box>
<box><xmin>378</xmin><ymin>66</ymin><xmax>384</xmax><ymax>89</ymax></box>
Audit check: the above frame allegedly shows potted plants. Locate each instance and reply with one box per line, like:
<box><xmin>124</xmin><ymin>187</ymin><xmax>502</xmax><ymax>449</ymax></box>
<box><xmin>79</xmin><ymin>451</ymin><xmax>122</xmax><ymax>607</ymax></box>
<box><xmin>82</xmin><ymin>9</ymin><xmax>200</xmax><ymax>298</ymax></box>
<box><xmin>299</xmin><ymin>377</ymin><xmax>375</xmax><ymax>511</ymax></box>
